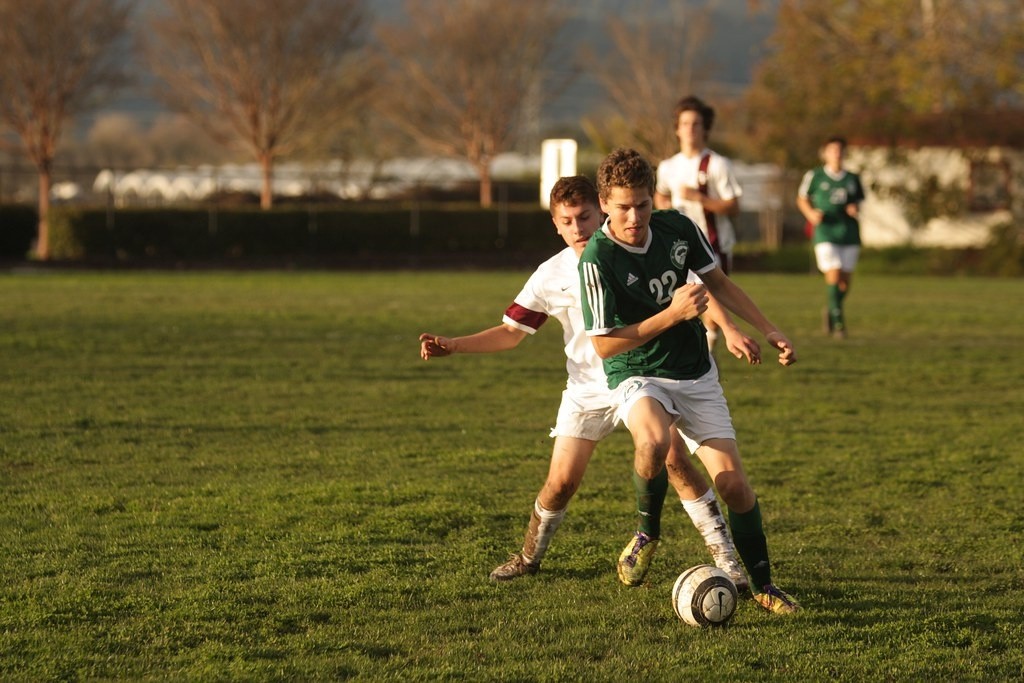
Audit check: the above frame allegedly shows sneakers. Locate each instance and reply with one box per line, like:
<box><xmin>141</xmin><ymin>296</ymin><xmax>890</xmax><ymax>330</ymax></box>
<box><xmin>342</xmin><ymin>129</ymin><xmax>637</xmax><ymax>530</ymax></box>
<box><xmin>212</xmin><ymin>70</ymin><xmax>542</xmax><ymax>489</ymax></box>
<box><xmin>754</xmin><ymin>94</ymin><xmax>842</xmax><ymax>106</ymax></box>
<box><xmin>617</xmin><ymin>530</ymin><xmax>661</xmax><ymax>587</ymax></box>
<box><xmin>716</xmin><ymin>559</ymin><xmax>748</xmax><ymax>591</ymax></box>
<box><xmin>489</xmin><ymin>553</ymin><xmax>541</xmax><ymax>583</ymax></box>
<box><xmin>751</xmin><ymin>583</ymin><xmax>805</xmax><ymax>620</ymax></box>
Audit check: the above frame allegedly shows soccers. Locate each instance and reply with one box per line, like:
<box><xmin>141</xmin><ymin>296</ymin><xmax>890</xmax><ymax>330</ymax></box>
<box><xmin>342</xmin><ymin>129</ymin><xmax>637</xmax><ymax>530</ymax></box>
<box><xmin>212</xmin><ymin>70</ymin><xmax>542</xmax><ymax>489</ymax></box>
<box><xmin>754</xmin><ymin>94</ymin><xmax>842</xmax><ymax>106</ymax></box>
<box><xmin>671</xmin><ymin>562</ymin><xmax>739</xmax><ymax>630</ymax></box>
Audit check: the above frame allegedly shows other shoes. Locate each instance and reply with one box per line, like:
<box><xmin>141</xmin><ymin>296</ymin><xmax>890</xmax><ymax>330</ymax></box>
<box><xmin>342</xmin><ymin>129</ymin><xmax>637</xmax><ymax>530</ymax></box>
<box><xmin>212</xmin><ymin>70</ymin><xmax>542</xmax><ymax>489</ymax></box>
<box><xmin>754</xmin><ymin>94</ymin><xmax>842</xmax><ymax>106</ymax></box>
<box><xmin>821</xmin><ymin>305</ymin><xmax>846</xmax><ymax>340</ymax></box>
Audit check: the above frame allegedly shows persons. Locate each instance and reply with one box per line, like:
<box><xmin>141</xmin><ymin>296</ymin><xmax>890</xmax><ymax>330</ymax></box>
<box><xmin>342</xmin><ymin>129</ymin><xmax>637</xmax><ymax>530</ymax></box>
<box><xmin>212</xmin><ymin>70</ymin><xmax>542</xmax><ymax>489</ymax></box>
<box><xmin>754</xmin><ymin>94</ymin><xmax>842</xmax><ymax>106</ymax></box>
<box><xmin>796</xmin><ymin>134</ymin><xmax>866</xmax><ymax>339</ymax></box>
<box><xmin>653</xmin><ymin>95</ymin><xmax>743</xmax><ymax>352</ymax></box>
<box><xmin>578</xmin><ymin>148</ymin><xmax>804</xmax><ymax>617</ymax></box>
<box><xmin>420</xmin><ymin>176</ymin><xmax>750</xmax><ymax>589</ymax></box>
